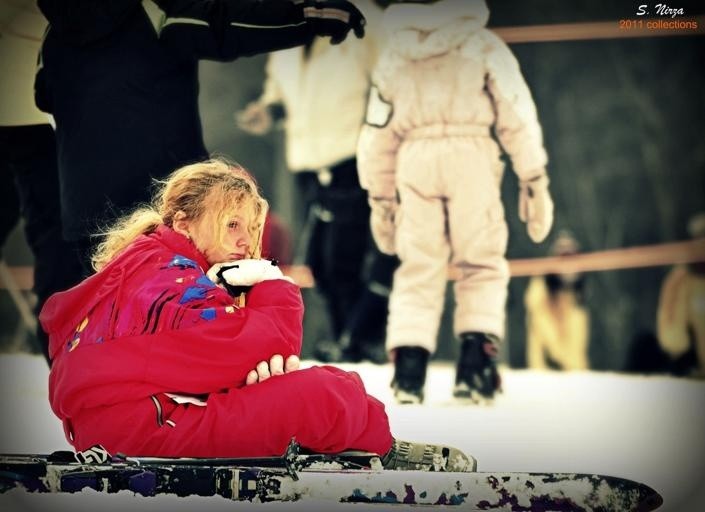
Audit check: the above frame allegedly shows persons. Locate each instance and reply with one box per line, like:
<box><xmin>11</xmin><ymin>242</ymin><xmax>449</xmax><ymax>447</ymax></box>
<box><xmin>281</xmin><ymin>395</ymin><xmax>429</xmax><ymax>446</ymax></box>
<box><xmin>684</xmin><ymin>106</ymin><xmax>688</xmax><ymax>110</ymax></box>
<box><xmin>355</xmin><ymin>0</ymin><xmax>556</xmax><ymax>408</ymax></box>
<box><xmin>30</xmin><ymin>0</ymin><xmax>368</xmax><ymax>287</ymax></box>
<box><xmin>38</xmin><ymin>153</ymin><xmax>480</xmax><ymax>476</ymax></box>
<box><xmin>655</xmin><ymin>212</ymin><xmax>705</xmax><ymax>378</ymax></box>
<box><xmin>0</xmin><ymin>0</ymin><xmax>59</xmax><ymax>375</ymax></box>
<box><xmin>522</xmin><ymin>229</ymin><xmax>593</xmax><ymax>372</ymax></box>
<box><xmin>228</xmin><ymin>20</ymin><xmax>404</xmax><ymax>364</ymax></box>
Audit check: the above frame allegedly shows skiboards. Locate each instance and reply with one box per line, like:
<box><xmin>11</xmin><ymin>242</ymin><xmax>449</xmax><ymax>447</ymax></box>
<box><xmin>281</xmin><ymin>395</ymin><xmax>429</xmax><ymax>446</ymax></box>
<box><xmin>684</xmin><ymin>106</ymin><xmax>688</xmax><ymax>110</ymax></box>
<box><xmin>1</xmin><ymin>448</ymin><xmax>662</xmax><ymax>512</ymax></box>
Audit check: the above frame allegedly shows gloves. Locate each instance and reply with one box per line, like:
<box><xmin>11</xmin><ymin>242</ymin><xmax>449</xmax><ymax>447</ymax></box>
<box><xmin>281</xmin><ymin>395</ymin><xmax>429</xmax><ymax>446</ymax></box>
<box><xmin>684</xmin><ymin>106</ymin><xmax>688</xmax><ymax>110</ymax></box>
<box><xmin>513</xmin><ymin>174</ymin><xmax>557</xmax><ymax>246</ymax></box>
<box><xmin>298</xmin><ymin>0</ymin><xmax>366</xmax><ymax>47</ymax></box>
<box><xmin>204</xmin><ymin>252</ymin><xmax>284</xmax><ymax>298</ymax></box>
<box><xmin>245</xmin><ymin>350</ymin><xmax>305</xmax><ymax>385</ymax></box>
<box><xmin>366</xmin><ymin>196</ymin><xmax>402</xmax><ymax>259</ymax></box>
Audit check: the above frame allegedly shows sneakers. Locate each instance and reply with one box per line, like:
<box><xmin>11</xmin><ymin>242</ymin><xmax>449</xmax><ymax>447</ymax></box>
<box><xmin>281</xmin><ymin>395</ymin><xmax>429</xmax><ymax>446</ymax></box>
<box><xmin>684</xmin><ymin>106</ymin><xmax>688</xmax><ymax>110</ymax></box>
<box><xmin>450</xmin><ymin>332</ymin><xmax>503</xmax><ymax>405</ymax></box>
<box><xmin>381</xmin><ymin>437</ymin><xmax>478</xmax><ymax>475</ymax></box>
<box><xmin>389</xmin><ymin>343</ymin><xmax>428</xmax><ymax>404</ymax></box>
